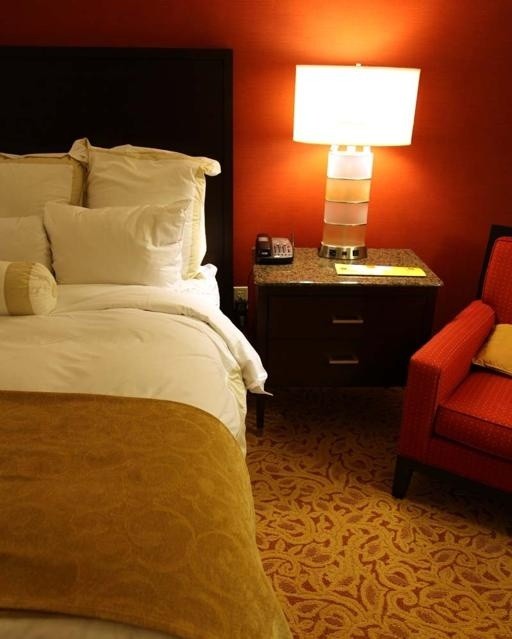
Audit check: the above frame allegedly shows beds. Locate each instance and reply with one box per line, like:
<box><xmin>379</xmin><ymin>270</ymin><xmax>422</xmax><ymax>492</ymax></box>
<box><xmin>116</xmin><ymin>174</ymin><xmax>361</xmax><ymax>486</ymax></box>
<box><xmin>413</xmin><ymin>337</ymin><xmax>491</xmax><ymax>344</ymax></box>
<box><xmin>0</xmin><ymin>38</ymin><xmax>299</xmax><ymax>639</ymax></box>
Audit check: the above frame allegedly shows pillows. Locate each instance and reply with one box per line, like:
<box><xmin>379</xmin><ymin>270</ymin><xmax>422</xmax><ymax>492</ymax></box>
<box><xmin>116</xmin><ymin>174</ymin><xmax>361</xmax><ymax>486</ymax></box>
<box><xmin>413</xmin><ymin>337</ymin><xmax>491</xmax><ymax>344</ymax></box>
<box><xmin>68</xmin><ymin>135</ymin><xmax>222</xmax><ymax>283</ymax></box>
<box><xmin>1</xmin><ymin>148</ymin><xmax>86</xmax><ymax>219</ymax></box>
<box><xmin>472</xmin><ymin>319</ymin><xmax>512</xmax><ymax>380</ymax></box>
<box><xmin>40</xmin><ymin>196</ymin><xmax>197</xmax><ymax>289</ymax></box>
<box><xmin>1</xmin><ymin>212</ymin><xmax>56</xmax><ymax>281</ymax></box>
<box><xmin>1</xmin><ymin>259</ymin><xmax>63</xmax><ymax>317</ymax></box>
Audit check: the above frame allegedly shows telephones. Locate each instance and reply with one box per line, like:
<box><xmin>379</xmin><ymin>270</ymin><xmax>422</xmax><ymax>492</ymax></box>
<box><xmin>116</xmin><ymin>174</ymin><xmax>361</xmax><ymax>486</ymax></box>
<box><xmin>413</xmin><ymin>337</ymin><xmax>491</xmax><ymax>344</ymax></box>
<box><xmin>255</xmin><ymin>233</ymin><xmax>294</xmax><ymax>265</ymax></box>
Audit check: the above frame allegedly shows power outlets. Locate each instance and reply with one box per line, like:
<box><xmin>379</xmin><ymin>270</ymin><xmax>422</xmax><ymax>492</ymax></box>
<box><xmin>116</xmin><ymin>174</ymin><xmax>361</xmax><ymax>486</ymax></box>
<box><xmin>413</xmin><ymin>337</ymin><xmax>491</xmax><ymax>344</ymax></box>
<box><xmin>234</xmin><ymin>283</ymin><xmax>250</xmax><ymax>306</ymax></box>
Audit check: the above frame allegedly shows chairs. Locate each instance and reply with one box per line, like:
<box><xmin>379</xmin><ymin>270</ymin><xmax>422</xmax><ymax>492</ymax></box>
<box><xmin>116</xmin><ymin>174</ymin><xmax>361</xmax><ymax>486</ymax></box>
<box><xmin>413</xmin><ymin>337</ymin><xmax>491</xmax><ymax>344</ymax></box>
<box><xmin>393</xmin><ymin>221</ymin><xmax>512</xmax><ymax>512</ymax></box>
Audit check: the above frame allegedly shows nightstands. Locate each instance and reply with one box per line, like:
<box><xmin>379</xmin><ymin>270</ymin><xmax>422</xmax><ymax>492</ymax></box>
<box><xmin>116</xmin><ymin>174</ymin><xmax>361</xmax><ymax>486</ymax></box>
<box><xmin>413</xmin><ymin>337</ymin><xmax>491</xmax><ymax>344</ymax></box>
<box><xmin>246</xmin><ymin>241</ymin><xmax>447</xmax><ymax>443</ymax></box>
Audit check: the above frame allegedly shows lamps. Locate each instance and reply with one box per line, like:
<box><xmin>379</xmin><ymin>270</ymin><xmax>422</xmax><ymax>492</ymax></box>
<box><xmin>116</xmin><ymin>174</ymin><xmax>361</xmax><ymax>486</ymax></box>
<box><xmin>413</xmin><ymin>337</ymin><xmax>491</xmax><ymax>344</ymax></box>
<box><xmin>290</xmin><ymin>57</ymin><xmax>429</xmax><ymax>262</ymax></box>
